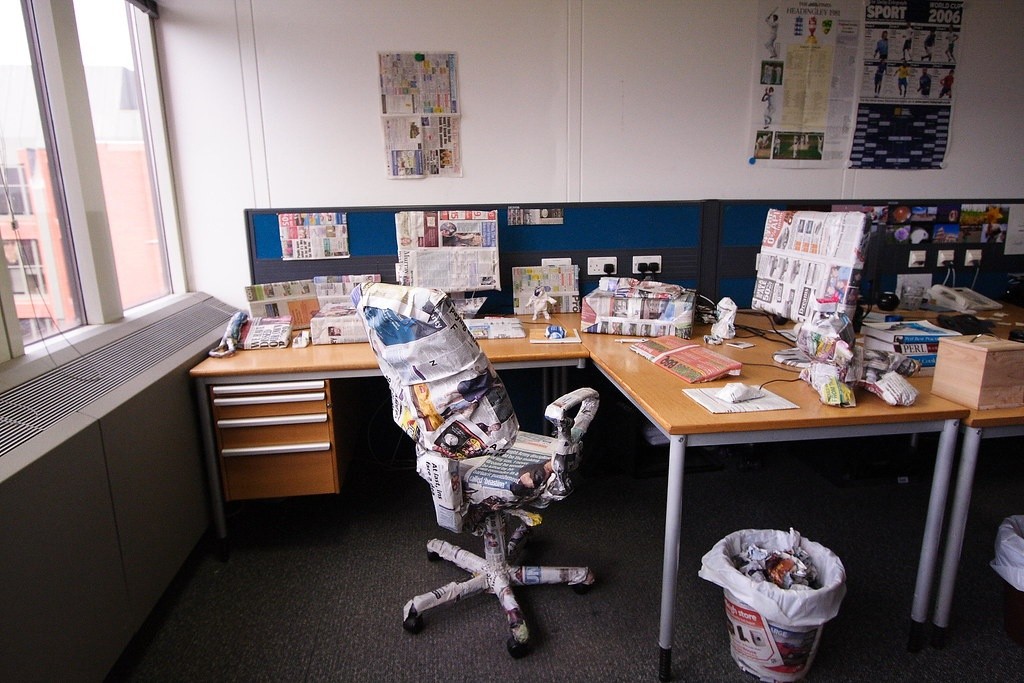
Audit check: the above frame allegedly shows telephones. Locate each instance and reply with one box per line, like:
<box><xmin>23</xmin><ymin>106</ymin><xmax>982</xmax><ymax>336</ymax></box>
<box><xmin>209</xmin><ymin>313</ymin><xmax>294</xmax><ymax>358</ymax></box>
<box><xmin>926</xmin><ymin>284</ymin><xmax>1003</xmax><ymax>314</ymax></box>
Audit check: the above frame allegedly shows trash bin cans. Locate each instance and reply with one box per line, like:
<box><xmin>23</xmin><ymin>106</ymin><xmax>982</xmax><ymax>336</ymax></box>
<box><xmin>996</xmin><ymin>515</ymin><xmax>1024</xmax><ymax>648</ymax></box>
<box><xmin>721</xmin><ymin>529</ymin><xmax>844</xmax><ymax>683</ymax></box>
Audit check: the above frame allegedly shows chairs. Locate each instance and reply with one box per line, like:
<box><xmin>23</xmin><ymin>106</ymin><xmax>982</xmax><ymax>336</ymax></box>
<box><xmin>351</xmin><ymin>282</ymin><xmax>601</xmax><ymax>658</ymax></box>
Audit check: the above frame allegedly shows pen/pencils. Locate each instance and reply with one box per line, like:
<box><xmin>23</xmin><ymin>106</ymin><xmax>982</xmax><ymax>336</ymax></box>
<box><xmin>615</xmin><ymin>338</ymin><xmax>647</xmax><ymax>343</ymax></box>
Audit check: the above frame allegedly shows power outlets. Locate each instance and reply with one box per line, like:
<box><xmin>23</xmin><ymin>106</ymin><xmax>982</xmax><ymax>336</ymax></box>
<box><xmin>908</xmin><ymin>251</ymin><xmax>926</xmax><ymax>267</ymax></box>
<box><xmin>587</xmin><ymin>256</ymin><xmax>617</xmax><ymax>275</ymax></box>
<box><xmin>937</xmin><ymin>250</ymin><xmax>954</xmax><ymax>267</ymax></box>
<box><xmin>964</xmin><ymin>250</ymin><xmax>982</xmax><ymax>267</ymax></box>
<box><xmin>541</xmin><ymin>258</ymin><xmax>571</xmax><ymax>265</ymax></box>
<box><xmin>633</xmin><ymin>255</ymin><xmax>662</xmax><ymax>273</ymax></box>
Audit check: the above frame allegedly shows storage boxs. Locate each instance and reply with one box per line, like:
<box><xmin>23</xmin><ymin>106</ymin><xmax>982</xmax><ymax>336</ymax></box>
<box><xmin>311</xmin><ymin>303</ymin><xmax>369</xmax><ymax>345</ymax></box>
<box><xmin>931</xmin><ymin>333</ymin><xmax>1024</xmax><ymax>410</ymax></box>
<box><xmin>580</xmin><ymin>285</ymin><xmax>697</xmax><ymax>339</ymax></box>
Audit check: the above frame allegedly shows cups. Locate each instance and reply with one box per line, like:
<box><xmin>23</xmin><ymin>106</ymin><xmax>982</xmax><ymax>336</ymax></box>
<box><xmin>901</xmin><ymin>285</ymin><xmax>924</xmax><ymax>310</ymax></box>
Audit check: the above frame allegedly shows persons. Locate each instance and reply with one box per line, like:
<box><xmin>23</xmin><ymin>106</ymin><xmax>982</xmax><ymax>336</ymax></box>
<box><xmin>474</xmin><ymin>423</ymin><xmax>502</xmax><ymax>436</ymax></box>
<box><xmin>295</xmin><ymin>215</ymin><xmax>304</xmax><ymax>226</ymax></box>
<box><xmin>514</xmin><ymin>461</ymin><xmax>554</xmax><ymax>496</ymax></box>
<box><xmin>755</xmin><ymin>14</ymin><xmax>960</xmax><ymax>160</ymax></box>
<box><xmin>524</xmin><ymin>285</ymin><xmax>556</xmax><ymax>321</ymax></box>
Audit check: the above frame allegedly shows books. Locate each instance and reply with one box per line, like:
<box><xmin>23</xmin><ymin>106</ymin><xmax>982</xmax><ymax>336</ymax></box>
<box><xmin>860</xmin><ymin>320</ymin><xmax>962</xmax><ymax>378</ymax></box>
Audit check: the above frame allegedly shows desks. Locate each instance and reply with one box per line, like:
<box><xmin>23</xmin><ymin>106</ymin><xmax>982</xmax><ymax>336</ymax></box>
<box><xmin>190</xmin><ymin>312</ymin><xmax>590</xmax><ymax>545</ymax></box>
<box><xmin>553</xmin><ymin>299</ymin><xmax>1024</xmax><ymax>683</ymax></box>
